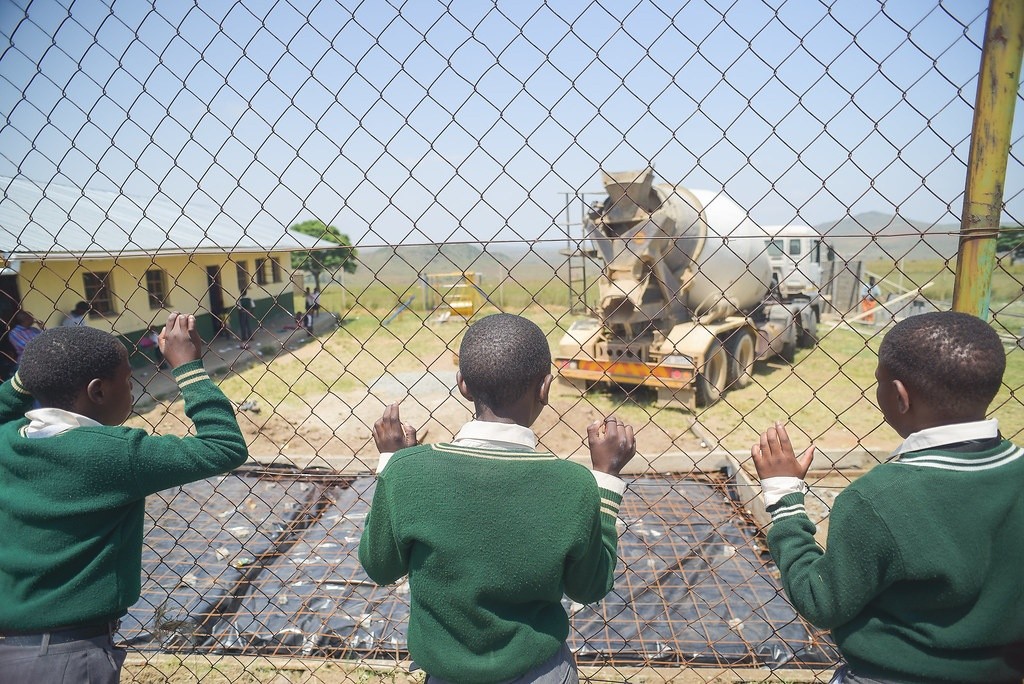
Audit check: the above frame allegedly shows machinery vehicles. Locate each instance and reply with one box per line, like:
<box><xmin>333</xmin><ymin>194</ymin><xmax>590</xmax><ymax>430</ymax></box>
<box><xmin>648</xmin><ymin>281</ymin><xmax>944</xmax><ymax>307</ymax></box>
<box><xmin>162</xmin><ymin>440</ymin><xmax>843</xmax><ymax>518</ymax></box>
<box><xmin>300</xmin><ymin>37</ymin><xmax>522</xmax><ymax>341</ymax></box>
<box><xmin>556</xmin><ymin>167</ymin><xmax>836</xmax><ymax>412</ymax></box>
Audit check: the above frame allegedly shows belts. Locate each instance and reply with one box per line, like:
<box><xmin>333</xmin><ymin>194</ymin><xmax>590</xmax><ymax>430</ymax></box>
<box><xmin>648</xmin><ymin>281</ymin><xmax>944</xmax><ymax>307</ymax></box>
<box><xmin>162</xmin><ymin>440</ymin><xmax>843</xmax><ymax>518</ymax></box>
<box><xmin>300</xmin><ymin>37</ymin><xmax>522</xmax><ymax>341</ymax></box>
<box><xmin>0</xmin><ymin>619</ymin><xmax>122</xmax><ymax>646</ymax></box>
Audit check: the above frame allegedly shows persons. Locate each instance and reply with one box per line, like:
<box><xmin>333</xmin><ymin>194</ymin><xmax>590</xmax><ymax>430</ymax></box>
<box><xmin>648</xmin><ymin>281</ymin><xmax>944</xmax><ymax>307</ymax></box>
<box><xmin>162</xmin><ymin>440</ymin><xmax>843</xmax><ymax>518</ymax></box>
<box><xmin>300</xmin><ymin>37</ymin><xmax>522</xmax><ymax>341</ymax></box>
<box><xmin>750</xmin><ymin>312</ymin><xmax>1024</xmax><ymax>684</ymax></box>
<box><xmin>861</xmin><ymin>278</ymin><xmax>886</xmax><ymax>328</ymax></box>
<box><xmin>359</xmin><ymin>311</ymin><xmax>636</xmax><ymax>684</ymax></box>
<box><xmin>12</xmin><ymin>287</ymin><xmax>319</xmax><ymax>413</ymax></box>
<box><xmin>0</xmin><ymin>308</ymin><xmax>247</xmax><ymax>684</ymax></box>
